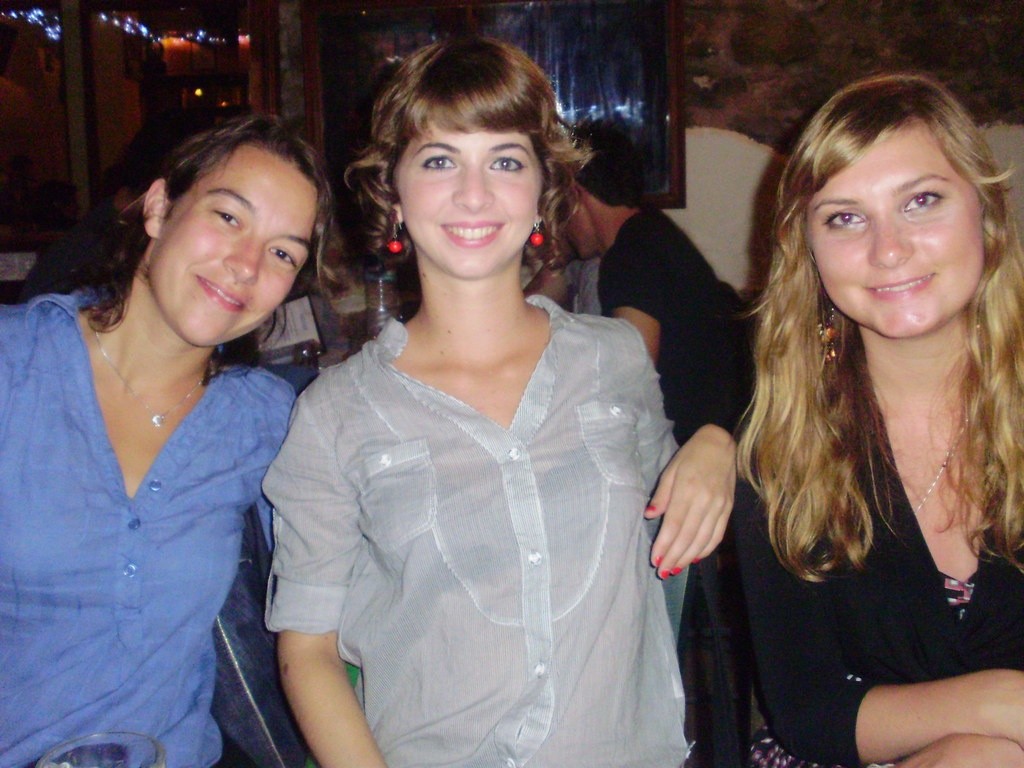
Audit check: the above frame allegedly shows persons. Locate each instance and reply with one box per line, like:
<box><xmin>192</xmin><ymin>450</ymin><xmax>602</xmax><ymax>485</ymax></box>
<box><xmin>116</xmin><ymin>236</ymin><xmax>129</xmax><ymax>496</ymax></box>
<box><xmin>527</xmin><ymin>120</ymin><xmax>755</xmax><ymax>443</ymax></box>
<box><xmin>262</xmin><ymin>36</ymin><xmax>740</xmax><ymax>768</ymax></box>
<box><xmin>732</xmin><ymin>73</ymin><xmax>1024</xmax><ymax>768</ymax></box>
<box><xmin>0</xmin><ymin>108</ymin><xmax>328</xmax><ymax>768</ymax></box>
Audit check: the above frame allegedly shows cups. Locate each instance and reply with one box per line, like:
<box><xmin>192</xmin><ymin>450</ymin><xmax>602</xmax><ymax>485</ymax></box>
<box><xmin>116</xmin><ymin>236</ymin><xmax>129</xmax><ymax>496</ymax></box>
<box><xmin>35</xmin><ymin>731</ymin><xmax>167</xmax><ymax>768</ymax></box>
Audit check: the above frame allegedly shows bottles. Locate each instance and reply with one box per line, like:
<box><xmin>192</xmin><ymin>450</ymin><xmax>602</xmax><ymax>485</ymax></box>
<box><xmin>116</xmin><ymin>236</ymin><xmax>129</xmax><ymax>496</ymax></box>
<box><xmin>363</xmin><ymin>255</ymin><xmax>398</xmax><ymax>341</ymax></box>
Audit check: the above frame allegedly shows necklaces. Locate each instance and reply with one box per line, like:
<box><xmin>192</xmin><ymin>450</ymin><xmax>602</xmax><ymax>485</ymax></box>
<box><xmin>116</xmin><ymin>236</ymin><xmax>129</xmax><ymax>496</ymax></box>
<box><xmin>96</xmin><ymin>328</ymin><xmax>205</xmax><ymax>427</ymax></box>
<box><xmin>912</xmin><ymin>413</ymin><xmax>971</xmax><ymax>515</ymax></box>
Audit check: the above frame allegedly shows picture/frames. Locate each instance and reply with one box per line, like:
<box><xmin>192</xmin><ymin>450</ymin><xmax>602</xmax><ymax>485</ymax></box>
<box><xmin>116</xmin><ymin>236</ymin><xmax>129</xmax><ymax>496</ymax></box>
<box><xmin>303</xmin><ymin>0</ymin><xmax>688</xmax><ymax>209</ymax></box>
